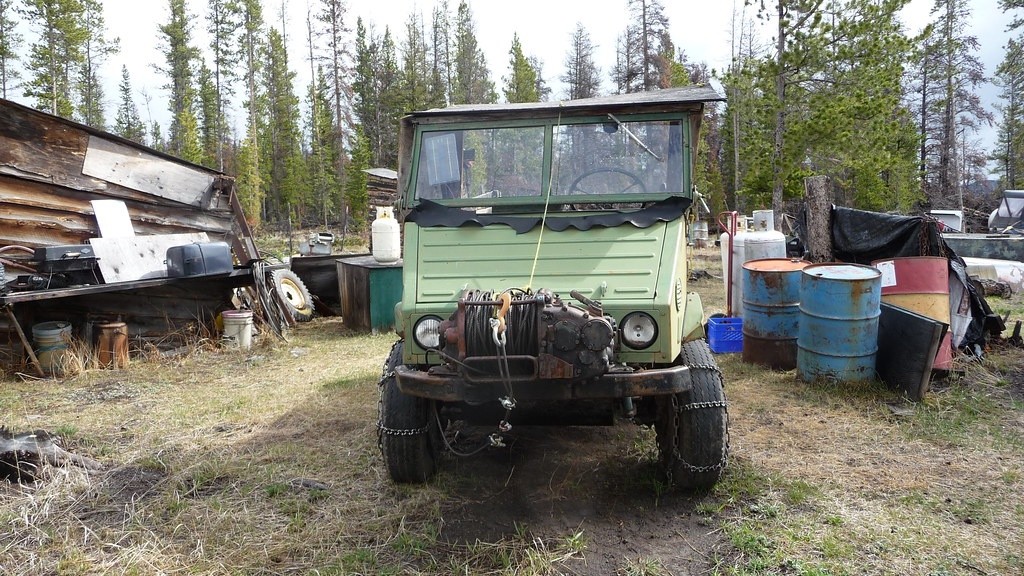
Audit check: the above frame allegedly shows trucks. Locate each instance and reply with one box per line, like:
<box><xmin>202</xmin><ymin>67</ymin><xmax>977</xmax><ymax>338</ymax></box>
<box><xmin>376</xmin><ymin>80</ymin><xmax>731</xmax><ymax>492</ymax></box>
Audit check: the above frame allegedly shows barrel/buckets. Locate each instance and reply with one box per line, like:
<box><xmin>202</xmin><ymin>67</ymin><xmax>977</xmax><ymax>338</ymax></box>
<box><xmin>870</xmin><ymin>256</ymin><xmax>954</xmax><ymax>371</ymax></box>
<box><xmin>741</xmin><ymin>257</ymin><xmax>813</xmax><ymax>371</ymax></box>
<box><xmin>221</xmin><ymin>310</ymin><xmax>253</xmax><ymax>351</ymax></box>
<box><xmin>692</xmin><ymin>219</ymin><xmax>708</xmax><ymax>247</ymax></box>
<box><xmin>32</xmin><ymin>321</ymin><xmax>72</xmax><ymax>373</ymax></box>
<box><xmin>92</xmin><ymin>323</ymin><xmax>129</xmax><ymax>370</ymax></box>
<box><xmin>796</xmin><ymin>263</ymin><xmax>882</xmax><ymax>388</ymax></box>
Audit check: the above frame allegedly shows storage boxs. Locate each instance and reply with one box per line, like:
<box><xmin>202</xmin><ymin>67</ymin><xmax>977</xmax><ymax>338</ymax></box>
<box><xmin>706</xmin><ymin>318</ymin><xmax>743</xmax><ymax>354</ymax></box>
<box><xmin>335</xmin><ymin>255</ymin><xmax>404</xmax><ymax>334</ymax></box>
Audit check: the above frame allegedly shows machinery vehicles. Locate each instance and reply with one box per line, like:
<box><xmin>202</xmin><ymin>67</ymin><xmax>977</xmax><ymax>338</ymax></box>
<box><xmin>988</xmin><ymin>190</ymin><xmax>1024</xmax><ymax>237</ymax></box>
